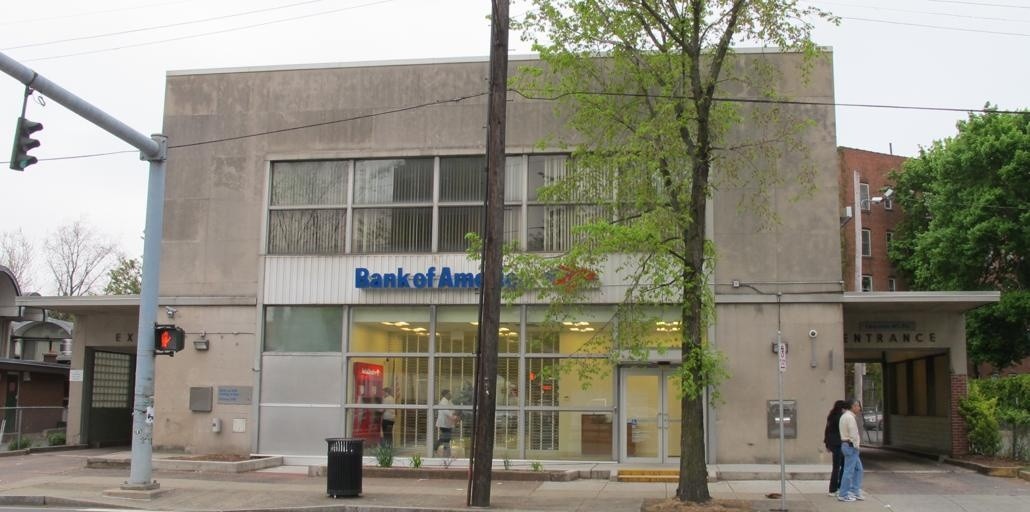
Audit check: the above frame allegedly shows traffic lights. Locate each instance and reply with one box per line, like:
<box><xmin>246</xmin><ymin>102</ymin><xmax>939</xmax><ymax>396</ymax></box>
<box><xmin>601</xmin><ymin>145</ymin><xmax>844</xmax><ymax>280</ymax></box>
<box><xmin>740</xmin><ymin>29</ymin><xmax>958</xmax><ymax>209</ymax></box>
<box><xmin>10</xmin><ymin>116</ymin><xmax>43</xmax><ymax>172</ymax></box>
<box><xmin>155</xmin><ymin>326</ymin><xmax>185</xmax><ymax>353</ymax></box>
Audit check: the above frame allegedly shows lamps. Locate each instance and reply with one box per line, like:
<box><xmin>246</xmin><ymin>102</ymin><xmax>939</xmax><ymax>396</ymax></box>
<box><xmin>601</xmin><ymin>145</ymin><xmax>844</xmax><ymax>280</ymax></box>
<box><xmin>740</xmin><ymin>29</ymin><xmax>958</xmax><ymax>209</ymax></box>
<box><xmin>193</xmin><ymin>338</ymin><xmax>210</xmax><ymax>350</ymax></box>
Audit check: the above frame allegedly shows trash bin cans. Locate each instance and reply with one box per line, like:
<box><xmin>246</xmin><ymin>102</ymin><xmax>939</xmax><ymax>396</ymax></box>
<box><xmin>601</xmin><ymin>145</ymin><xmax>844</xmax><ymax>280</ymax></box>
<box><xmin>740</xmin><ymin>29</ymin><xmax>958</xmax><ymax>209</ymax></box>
<box><xmin>325</xmin><ymin>438</ymin><xmax>364</xmax><ymax>498</ymax></box>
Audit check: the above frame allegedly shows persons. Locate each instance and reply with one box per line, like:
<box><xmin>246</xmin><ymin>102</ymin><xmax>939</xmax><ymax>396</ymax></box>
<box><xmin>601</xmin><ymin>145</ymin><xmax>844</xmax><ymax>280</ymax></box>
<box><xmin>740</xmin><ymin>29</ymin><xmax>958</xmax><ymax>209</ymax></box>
<box><xmin>433</xmin><ymin>390</ymin><xmax>458</xmax><ymax>457</ymax></box>
<box><xmin>376</xmin><ymin>388</ymin><xmax>395</xmax><ymax>444</ymax></box>
<box><xmin>824</xmin><ymin>399</ymin><xmax>865</xmax><ymax>501</ymax></box>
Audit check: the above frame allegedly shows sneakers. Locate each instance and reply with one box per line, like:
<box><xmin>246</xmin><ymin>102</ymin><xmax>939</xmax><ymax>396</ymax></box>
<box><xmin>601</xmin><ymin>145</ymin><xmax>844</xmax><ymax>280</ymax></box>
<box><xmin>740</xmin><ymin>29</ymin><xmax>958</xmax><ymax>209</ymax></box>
<box><xmin>827</xmin><ymin>492</ymin><xmax>865</xmax><ymax>502</ymax></box>
